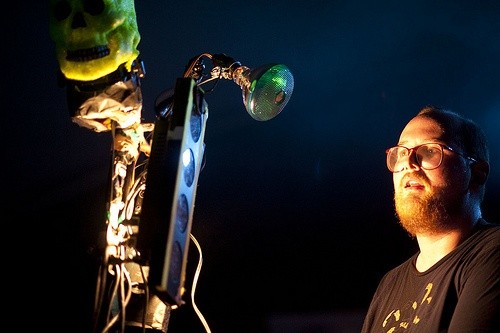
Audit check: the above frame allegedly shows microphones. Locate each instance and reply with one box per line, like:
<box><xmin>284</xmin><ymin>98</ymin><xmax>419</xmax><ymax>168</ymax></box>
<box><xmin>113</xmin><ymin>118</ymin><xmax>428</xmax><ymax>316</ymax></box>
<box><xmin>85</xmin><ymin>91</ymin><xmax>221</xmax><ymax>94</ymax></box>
<box><xmin>211</xmin><ymin>51</ymin><xmax>295</xmax><ymax>121</ymax></box>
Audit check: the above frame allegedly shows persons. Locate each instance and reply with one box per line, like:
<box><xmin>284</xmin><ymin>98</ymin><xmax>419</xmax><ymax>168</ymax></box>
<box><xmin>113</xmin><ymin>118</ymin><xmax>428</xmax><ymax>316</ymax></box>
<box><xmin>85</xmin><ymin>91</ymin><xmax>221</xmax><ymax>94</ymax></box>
<box><xmin>359</xmin><ymin>108</ymin><xmax>499</xmax><ymax>333</ymax></box>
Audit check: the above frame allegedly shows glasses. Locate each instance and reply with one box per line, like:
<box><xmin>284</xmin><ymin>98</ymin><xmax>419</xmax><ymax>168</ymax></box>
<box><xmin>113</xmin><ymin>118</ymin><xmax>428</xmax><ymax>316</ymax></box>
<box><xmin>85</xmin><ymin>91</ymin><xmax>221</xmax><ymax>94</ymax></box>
<box><xmin>386</xmin><ymin>142</ymin><xmax>479</xmax><ymax>172</ymax></box>
